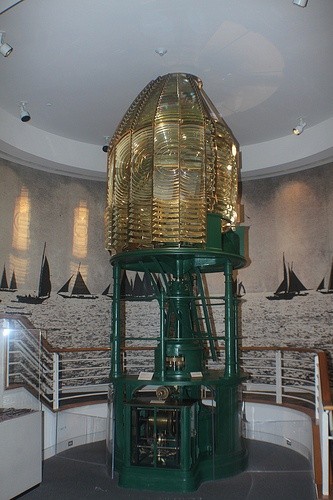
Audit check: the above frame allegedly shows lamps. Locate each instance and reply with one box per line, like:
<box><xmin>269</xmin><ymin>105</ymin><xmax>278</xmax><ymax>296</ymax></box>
<box><xmin>0</xmin><ymin>31</ymin><xmax>13</xmax><ymax>57</ymax></box>
<box><xmin>293</xmin><ymin>0</ymin><xmax>308</xmax><ymax>7</ymax></box>
<box><xmin>293</xmin><ymin>118</ymin><xmax>307</xmax><ymax>135</ymax></box>
<box><xmin>18</xmin><ymin>100</ymin><xmax>31</xmax><ymax>122</ymax></box>
